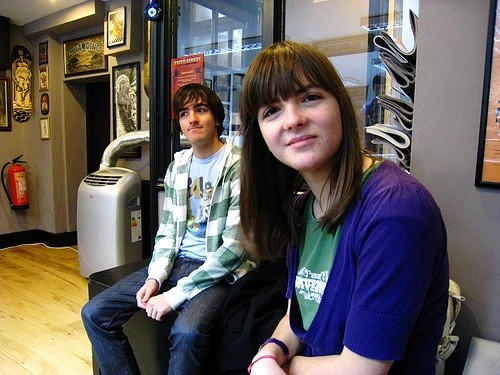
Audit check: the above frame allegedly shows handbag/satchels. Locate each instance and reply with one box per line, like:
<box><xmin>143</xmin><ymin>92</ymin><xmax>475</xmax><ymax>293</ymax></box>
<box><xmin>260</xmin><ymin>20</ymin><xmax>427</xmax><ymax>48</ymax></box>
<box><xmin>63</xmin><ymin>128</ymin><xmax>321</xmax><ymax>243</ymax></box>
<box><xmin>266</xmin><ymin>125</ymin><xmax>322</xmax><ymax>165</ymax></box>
<box><xmin>436</xmin><ymin>280</ymin><xmax>465</xmax><ymax>375</ymax></box>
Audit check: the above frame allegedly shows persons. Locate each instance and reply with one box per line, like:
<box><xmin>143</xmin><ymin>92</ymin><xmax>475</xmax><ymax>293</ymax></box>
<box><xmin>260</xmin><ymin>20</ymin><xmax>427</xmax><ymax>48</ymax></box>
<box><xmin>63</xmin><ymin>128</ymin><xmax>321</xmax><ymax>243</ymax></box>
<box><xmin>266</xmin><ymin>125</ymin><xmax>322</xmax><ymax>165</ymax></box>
<box><xmin>356</xmin><ymin>73</ymin><xmax>388</xmax><ymax>162</ymax></box>
<box><xmin>12</xmin><ymin>48</ymin><xmax>34</xmax><ymax>112</ymax></box>
<box><xmin>81</xmin><ymin>83</ymin><xmax>258</xmax><ymax>375</ymax></box>
<box><xmin>236</xmin><ymin>42</ymin><xmax>450</xmax><ymax>375</ymax></box>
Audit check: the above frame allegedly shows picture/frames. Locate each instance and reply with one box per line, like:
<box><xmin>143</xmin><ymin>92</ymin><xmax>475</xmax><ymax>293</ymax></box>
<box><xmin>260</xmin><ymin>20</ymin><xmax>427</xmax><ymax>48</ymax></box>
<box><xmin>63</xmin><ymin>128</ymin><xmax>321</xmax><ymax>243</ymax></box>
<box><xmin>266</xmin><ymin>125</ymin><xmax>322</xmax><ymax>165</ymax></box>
<box><xmin>0</xmin><ymin>76</ymin><xmax>12</xmax><ymax>132</ymax></box>
<box><xmin>37</xmin><ymin>66</ymin><xmax>49</xmax><ymax>91</ymax></box>
<box><xmin>108</xmin><ymin>6</ymin><xmax>125</xmax><ymax>47</ymax></box>
<box><xmin>39</xmin><ymin>117</ymin><xmax>51</xmax><ymax>140</ymax></box>
<box><xmin>62</xmin><ymin>31</ymin><xmax>108</xmax><ymax>77</ymax></box>
<box><xmin>475</xmin><ymin>0</ymin><xmax>500</xmax><ymax>188</ymax></box>
<box><xmin>39</xmin><ymin>41</ymin><xmax>48</xmax><ymax>65</ymax></box>
<box><xmin>112</xmin><ymin>61</ymin><xmax>141</xmax><ymax>159</ymax></box>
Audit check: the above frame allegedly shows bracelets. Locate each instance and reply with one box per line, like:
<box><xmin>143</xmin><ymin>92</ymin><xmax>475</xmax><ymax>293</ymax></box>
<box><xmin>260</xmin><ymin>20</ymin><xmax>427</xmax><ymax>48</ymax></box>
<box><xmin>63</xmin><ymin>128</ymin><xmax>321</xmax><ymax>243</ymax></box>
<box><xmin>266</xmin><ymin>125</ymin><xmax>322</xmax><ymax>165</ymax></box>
<box><xmin>258</xmin><ymin>339</ymin><xmax>290</xmax><ymax>365</ymax></box>
<box><xmin>247</xmin><ymin>355</ymin><xmax>282</xmax><ymax>374</ymax></box>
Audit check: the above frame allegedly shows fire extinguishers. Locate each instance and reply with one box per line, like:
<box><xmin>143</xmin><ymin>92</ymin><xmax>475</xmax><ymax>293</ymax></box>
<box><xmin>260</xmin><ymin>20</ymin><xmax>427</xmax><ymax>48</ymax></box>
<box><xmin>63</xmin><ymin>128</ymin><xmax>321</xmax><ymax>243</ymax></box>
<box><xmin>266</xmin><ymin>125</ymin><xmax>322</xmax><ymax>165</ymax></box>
<box><xmin>1</xmin><ymin>154</ymin><xmax>30</xmax><ymax>211</ymax></box>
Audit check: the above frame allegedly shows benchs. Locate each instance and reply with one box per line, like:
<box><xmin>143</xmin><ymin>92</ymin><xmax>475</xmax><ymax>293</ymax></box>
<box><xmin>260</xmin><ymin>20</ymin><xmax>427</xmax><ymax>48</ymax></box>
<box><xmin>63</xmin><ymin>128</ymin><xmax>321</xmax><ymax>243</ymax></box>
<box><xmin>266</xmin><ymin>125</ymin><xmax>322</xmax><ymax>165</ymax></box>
<box><xmin>89</xmin><ymin>261</ymin><xmax>181</xmax><ymax>375</ymax></box>
